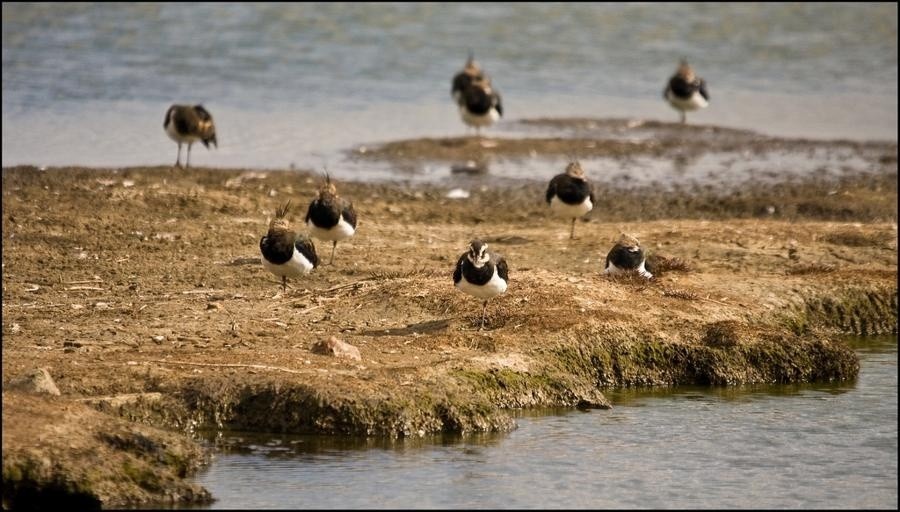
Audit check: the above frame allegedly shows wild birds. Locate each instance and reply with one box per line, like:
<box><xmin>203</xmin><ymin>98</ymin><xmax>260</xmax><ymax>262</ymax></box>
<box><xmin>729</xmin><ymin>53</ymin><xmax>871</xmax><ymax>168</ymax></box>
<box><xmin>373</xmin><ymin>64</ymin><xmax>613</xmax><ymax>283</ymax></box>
<box><xmin>545</xmin><ymin>161</ymin><xmax>596</xmax><ymax>239</ymax></box>
<box><xmin>663</xmin><ymin>55</ymin><xmax>710</xmax><ymax>124</ymax></box>
<box><xmin>604</xmin><ymin>233</ymin><xmax>653</xmax><ymax>281</ymax></box>
<box><xmin>259</xmin><ymin>198</ymin><xmax>322</xmax><ymax>294</ymax></box>
<box><xmin>450</xmin><ymin>55</ymin><xmax>503</xmax><ymax>136</ymax></box>
<box><xmin>162</xmin><ymin>104</ymin><xmax>217</xmax><ymax>170</ymax></box>
<box><xmin>452</xmin><ymin>238</ymin><xmax>509</xmax><ymax>333</ymax></box>
<box><xmin>303</xmin><ymin>167</ymin><xmax>357</xmax><ymax>268</ymax></box>
